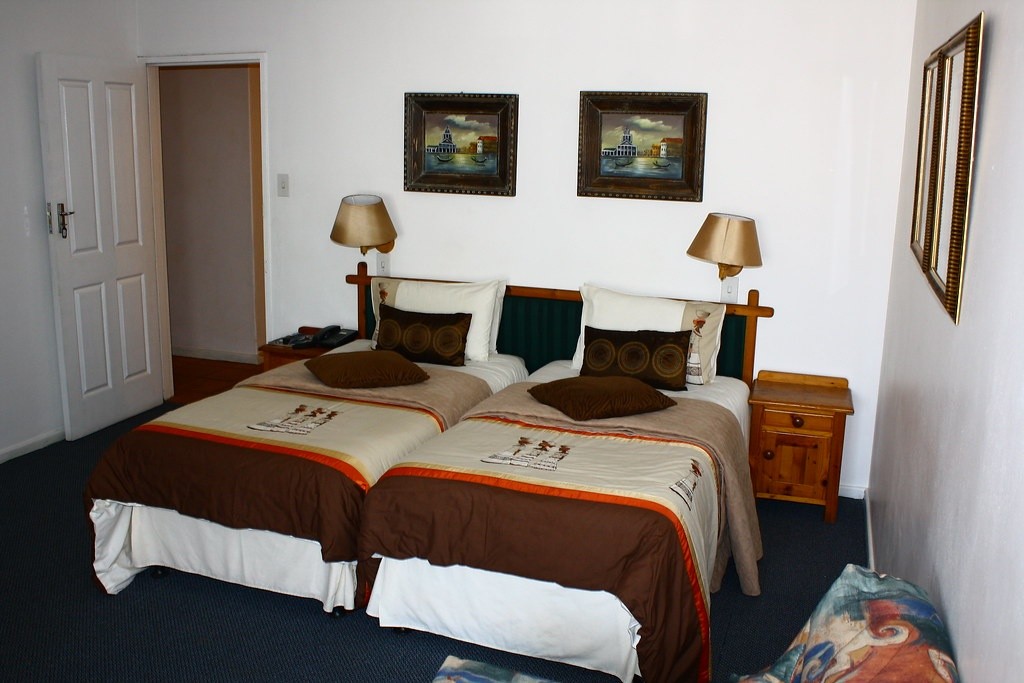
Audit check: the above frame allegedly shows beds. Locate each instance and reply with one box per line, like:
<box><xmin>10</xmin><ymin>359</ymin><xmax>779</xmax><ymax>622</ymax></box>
<box><xmin>84</xmin><ymin>274</ymin><xmax>528</xmax><ymax>613</ymax></box>
<box><xmin>357</xmin><ymin>289</ymin><xmax>775</xmax><ymax>683</ymax></box>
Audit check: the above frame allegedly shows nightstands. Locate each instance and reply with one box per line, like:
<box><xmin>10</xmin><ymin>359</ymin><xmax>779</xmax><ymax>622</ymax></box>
<box><xmin>258</xmin><ymin>326</ymin><xmax>322</xmax><ymax>373</ymax></box>
<box><xmin>748</xmin><ymin>370</ymin><xmax>854</xmax><ymax>526</ymax></box>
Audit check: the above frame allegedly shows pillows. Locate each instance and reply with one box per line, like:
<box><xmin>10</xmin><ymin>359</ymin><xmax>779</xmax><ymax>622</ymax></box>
<box><xmin>375</xmin><ymin>304</ymin><xmax>472</xmax><ymax>366</ymax></box>
<box><xmin>304</xmin><ymin>350</ymin><xmax>430</xmax><ymax>389</ymax></box>
<box><xmin>371</xmin><ymin>277</ymin><xmax>498</xmax><ymax>361</ymax></box>
<box><xmin>488</xmin><ymin>281</ymin><xmax>506</xmax><ymax>355</ymax></box>
<box><xmin>527</xmin><ymin>375</ymin><xmax>677</xmax><ymax>421</ymax></box>
<box><xmin>579</xmin><ymin>326</ymin><xmax>692</xmax><ymax>391</ymax></box>
<box><xmin>573</xmin><ymin>283</ymin><xmax>726</xmax><ymax>384</ymax></box>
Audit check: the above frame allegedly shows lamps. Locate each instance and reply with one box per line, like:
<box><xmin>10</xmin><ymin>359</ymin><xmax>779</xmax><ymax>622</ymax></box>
<box><xmin>687</xmin><ymin>213</ymin><xmax>762</xmax><ymax>280</ymax></box>
<box><xmin>330</xmin><ymin>193</ymin><xmax>397</xmax><ymax>254</ymax></box>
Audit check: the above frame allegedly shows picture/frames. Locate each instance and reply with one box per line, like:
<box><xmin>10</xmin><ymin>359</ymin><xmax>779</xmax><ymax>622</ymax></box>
<box><xmin>403</xmin><ymin>92</ymin><xmax>519</xmax><ymax>197</ymax></box>
<box><xmin>576</xmin><ymin>91</ymin><xmax>708</xmax><ymax>202</ymax></box>
<box><xmin>910</xmin><ymin>11</ymin><xmax>983</xmax><ymax>326</ymax></box>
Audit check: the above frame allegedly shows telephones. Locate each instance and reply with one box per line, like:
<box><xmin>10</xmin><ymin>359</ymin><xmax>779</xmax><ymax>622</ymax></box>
<box><xmin>311</xmin><ymin>325</ymin><xmax>359</xmax><ymax>348</ymax></box>
